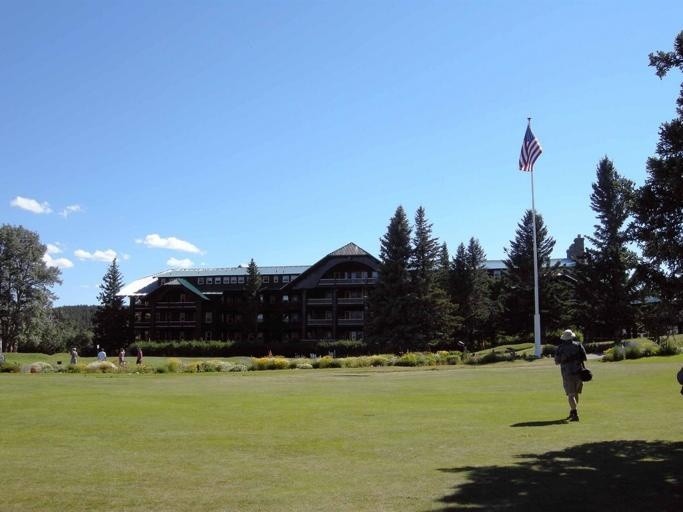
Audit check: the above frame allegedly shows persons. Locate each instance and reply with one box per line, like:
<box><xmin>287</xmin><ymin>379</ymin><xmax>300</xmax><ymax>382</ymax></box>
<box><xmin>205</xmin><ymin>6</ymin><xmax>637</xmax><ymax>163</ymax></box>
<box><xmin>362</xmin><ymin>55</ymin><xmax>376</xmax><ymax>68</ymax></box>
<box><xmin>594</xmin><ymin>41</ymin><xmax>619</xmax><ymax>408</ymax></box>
<box><xmin>70</xmin><ymin>347</ymin><xmax>78</xmax><ymax>364</ymax></box>
<box><xmin>676</xmin><ymin>366</ymin><xmax>683</xmax><ymax>395</ymax></box>
<box><xmin>0</xmin><ymin>336</ymin><xmax>19</xmax><ymax>353</ymax></box>
<box><xmin>135</xmin><ymin>347</ymin><xmax>142</xmax><ymax>364</ymax></box>
<box><xmin>118</xmin><ymin>347</ymin><xmax>125</xmax><ymax>364</ymax></box>
<box><xmin>97</xmin><ymin>348</ymin><xmax>106</xmax><ymax>361</ymax></box>
<box><xmin>554</xmin><ymin>329</ymin><xmax>587</xmax><ymax>421</ymax></box>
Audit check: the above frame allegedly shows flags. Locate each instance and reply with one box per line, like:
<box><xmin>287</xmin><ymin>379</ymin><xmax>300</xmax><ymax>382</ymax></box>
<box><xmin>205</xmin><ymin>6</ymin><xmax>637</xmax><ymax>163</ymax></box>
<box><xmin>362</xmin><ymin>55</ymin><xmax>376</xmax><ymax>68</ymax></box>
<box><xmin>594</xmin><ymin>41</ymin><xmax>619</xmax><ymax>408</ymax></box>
<box><xmin>517</xmin><ymin>125</ymin><xmax>542</xmax><ymax>173</ymax></box>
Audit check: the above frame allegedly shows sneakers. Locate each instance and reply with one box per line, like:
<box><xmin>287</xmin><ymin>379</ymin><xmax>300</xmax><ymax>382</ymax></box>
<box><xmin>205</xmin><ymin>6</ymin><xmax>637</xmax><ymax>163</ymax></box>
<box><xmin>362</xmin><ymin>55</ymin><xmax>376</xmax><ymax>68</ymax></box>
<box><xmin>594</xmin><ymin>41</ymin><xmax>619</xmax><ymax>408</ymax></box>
<box><xmin>568</xmin><ymin>414</ymin><xmax>579</xmax><ymax>421</ymax></box>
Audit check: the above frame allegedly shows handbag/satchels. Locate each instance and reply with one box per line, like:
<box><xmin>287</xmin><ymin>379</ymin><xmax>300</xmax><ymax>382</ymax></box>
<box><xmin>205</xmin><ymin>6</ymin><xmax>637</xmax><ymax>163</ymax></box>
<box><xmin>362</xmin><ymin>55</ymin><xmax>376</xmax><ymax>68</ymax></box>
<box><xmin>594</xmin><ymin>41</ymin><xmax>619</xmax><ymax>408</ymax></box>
<box><xmin>580</xmin><ymin>365</ymin><xmax>592</xmax><ymax>381</ymax></box>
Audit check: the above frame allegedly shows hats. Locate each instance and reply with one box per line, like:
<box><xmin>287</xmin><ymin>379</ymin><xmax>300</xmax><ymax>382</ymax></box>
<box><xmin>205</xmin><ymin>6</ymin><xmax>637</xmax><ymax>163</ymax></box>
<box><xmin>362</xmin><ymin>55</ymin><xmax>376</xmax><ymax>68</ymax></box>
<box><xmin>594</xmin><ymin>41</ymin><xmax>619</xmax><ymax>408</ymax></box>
<box><xmin>560</xmin><ymin>330</ymin><xmax>576</xmax><ymax>340</ymax></box>
